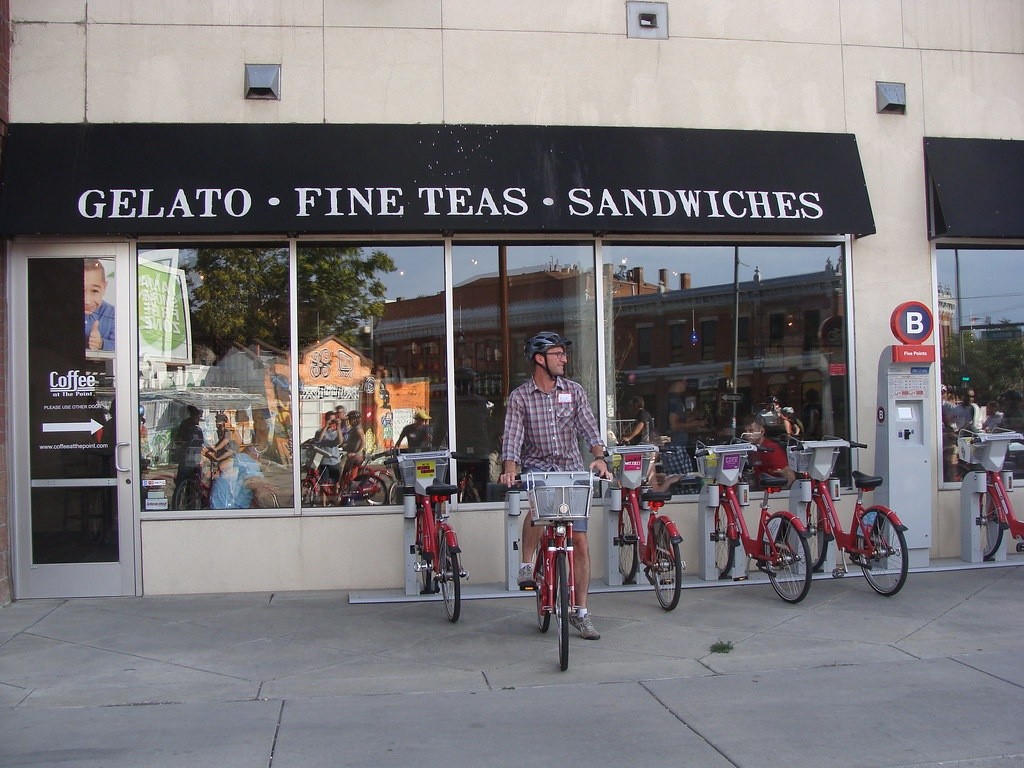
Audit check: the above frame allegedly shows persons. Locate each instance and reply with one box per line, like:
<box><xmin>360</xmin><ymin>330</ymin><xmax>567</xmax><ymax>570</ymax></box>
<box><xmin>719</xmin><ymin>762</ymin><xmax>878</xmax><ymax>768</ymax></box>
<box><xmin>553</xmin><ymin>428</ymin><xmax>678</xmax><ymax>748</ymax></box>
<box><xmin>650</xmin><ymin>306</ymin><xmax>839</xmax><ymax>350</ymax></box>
<box><xmin>85</xmin><ymin>258</ymin><xmax>115</xmax><ymax>351</ymax></box>
<box><xmin>315</xmin><ymin>405</ymin><xmax>366</xmax><ymax>507</ymax></box>
<box><xmin>623</xmin><ymin>396</ymin><xmax>654</xmax><ymax>445</ymax></box>
<box><xmin>500</xmin><ymin>332</ymin><xmax>614</xmax><ymax>640</ymax></box>
<box><xmin>941</xmin><ymin>384</ymin><xmax>1005</xmax><ymax>482</ymax></box>
<box><xmin>139</xmin><ymin>402</ymin><xmax>294</xmax><ymax>509</ymax></box>
<box><xmin>394</xmin><ymin>410</ymin><xmax>432</xmax><ymax>454</ymax></box>
<box><xmin>757</xmin><ymin>397</ymin><xmax>804</xmax><ymax>435</ymax></box>
<box><xmin>645</xmin><ymin>374</ymin><xmax>788</xmax><ymax>492</ymax></box>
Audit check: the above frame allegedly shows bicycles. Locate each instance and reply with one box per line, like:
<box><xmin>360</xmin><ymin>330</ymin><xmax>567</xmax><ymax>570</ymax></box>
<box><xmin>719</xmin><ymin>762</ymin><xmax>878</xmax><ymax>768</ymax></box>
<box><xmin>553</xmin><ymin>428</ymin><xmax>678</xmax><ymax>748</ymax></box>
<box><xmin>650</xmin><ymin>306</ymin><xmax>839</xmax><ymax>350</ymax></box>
<box><xmin>140</xmin><ymin>444</ymin><xmax>219</xmax><ymax>510</ymax></box>
<box><xmin>604</xmin><ymin>444</ymin><xmax>684</xmax><ymax>612</ymax></box>
<box><xmin>384</xmin><ymin>450</ymin><xmax>469</xmax><ymax>623</ymax></box>
<box><xmin>300</xmin><ymin>440</ymin><xmax>408</xmax><ymax>507</ymax></box>
<box><xmin>956</xmin><ymin>427</ymin><xmax>1024</xmax><ymax>561</ymax></box>
<box><xmin>692</xmin><ymin>438</ymin><xmax>813</xmax><ymax>603</ymax></box>
<box><xmin>452</xmin><ymin>453</ymin><xmax>485</xmax><ymax>504</ymax></box>
<box><xmin>497</xmin><ymin>469</ymin><xmax>613</xmax><ymax>670</ymax></box>
<box><xmin>773</xmin><ymin>431</ymin><xmax>910</xmax><ymax>596</ymax></box>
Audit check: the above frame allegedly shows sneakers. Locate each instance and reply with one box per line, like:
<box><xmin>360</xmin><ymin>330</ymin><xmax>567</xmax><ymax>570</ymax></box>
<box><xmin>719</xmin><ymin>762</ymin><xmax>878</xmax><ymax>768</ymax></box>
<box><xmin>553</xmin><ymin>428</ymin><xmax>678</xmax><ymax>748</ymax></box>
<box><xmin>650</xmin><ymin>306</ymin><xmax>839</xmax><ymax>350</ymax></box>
<box><xmin>569</xmin><ymin>607</ymin><xmax>600</xmax><ymax>640</ymax></box>
<box><xmin>517</xmin><ymin>565</ymin><xmax>533</xmax><ymax>586</ymax></box>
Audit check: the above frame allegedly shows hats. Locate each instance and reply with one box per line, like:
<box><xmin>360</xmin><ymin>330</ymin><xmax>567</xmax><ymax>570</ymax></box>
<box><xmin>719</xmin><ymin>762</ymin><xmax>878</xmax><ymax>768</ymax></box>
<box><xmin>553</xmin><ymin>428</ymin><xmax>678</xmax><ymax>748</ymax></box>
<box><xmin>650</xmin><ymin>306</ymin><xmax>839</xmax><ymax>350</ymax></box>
<box><xmin>782</xmin><ymin>407</ymin><xmax>794</xmax><ymax>414</ymax></box>
<box><xmin>414</xmin><ymin>412</ymin><xmax>431</xmax><ymax>420</ymax></box>
<box><xmin>179</xmin><ymin>405</ymin><xmax>203</xmax><ymax>420</ymax></box>
<box><xmin>941</xmin><ymin>383</ymin><xmax>947</xmax><ymax>392</ymax></box>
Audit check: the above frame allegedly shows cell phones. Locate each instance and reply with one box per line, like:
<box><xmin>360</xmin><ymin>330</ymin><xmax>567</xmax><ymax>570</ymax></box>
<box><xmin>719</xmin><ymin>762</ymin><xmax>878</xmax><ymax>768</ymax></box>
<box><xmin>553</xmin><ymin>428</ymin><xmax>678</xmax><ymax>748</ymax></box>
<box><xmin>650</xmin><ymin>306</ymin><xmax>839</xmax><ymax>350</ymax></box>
<box><xmin>331</xmin><ymin>419</ymin><xmax>338</xmax><ymax>423</ymax></box>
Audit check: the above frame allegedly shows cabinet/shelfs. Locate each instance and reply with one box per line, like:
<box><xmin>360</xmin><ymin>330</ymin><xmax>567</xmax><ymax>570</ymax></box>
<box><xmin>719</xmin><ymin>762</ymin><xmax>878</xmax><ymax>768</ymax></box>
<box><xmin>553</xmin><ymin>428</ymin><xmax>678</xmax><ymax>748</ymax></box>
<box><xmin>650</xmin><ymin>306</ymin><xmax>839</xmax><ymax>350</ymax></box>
<box><xmin>373</xmin><ymin>323</ymin><xmax>566</xmax><ymax>402</ymax></box>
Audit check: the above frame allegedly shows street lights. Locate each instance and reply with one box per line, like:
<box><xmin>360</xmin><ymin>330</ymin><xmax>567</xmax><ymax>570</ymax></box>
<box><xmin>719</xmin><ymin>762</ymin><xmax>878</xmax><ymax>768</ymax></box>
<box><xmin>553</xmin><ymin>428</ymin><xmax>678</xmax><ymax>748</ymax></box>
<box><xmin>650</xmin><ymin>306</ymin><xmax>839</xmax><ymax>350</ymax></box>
<box><xmin>731</xmin><ymin>260</ymin><xmax>760</xmax><ymax>436</ymax></box>
<box><xmin>363</xmin><ymin>315</ymin><xmax>374</xmax><ymax>362</ymax></box>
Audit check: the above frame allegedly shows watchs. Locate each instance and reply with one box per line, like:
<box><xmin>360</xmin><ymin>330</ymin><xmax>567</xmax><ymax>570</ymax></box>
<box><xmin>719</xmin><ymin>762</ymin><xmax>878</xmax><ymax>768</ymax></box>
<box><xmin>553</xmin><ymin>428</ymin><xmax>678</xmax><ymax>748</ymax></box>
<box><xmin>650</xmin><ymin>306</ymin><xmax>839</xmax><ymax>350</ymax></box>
<box><xmin>594</xmin><ymin>456</ymin><xmax>607</xmax><ymax>464</ymax></box>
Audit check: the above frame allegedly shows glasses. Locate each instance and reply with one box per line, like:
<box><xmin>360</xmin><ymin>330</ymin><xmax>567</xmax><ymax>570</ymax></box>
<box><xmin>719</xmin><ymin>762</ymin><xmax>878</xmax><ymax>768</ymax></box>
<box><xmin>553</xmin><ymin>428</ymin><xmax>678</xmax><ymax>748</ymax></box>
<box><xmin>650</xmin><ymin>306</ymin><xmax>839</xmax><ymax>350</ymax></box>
<box><xmin>967</xmin><ymin>395</ymin><xmax>975</xmax><ymax>398</ymax></box>
<box><xmin>544</xmin><ymin>352</ymin><xmax>567</xmax><ymax>359</ymax></box>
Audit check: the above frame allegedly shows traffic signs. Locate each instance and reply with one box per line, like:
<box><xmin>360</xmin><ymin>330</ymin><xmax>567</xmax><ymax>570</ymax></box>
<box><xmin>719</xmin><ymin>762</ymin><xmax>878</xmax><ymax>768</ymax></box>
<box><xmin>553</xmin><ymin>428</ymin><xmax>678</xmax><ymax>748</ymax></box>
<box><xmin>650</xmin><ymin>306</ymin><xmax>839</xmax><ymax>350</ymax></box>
<box><xmin>720</xmin><ymin>393</ymin><xmax>743</xmax><ymax>402</ymax></box>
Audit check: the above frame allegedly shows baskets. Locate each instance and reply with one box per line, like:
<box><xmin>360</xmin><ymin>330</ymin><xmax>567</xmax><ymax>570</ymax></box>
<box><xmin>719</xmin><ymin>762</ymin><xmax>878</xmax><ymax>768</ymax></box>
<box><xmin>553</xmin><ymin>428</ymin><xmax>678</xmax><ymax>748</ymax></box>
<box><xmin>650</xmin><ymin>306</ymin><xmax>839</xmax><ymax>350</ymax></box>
<box><xmin>696</xmin><ymin>440</ymin><xmax>751</xmax><ymax>477</ymax></box>
<box><xmin>399</xmin><ymin>450</ymin><xmax>448</xmax><ymax>487</ymax></box>
<box><xmin>958</xmin><ymin>427</ymin><xmax>1015</xmax><ymax>464</ymax></box>
<box><xmin>786</xmin><ymin>434</ymin><xmax>843</xmax><ymax>475</ymax></box>
<box><xmin>526</xmin><ymin>468</ymin><xmax>594</xmax><ymax>522</ymax></box>
<box><xmin>608</xmin><ymin>445</ymin><xmax>656</xmax><ymax>480</ymax></box>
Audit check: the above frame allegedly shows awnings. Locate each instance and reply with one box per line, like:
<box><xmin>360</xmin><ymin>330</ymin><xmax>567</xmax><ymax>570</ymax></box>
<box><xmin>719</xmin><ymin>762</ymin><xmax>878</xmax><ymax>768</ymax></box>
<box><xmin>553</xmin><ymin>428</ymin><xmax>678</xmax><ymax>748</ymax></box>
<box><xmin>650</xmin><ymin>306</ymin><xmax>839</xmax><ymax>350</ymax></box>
<box><xmin>0</xmin><ymin>123</ymin><xmax>878</xmax><ymax>242</ymax></box>
<box><xmin>924</xmin><ymin>136</ymin><xmax>1024</xmax><ymax>241</ymax></box>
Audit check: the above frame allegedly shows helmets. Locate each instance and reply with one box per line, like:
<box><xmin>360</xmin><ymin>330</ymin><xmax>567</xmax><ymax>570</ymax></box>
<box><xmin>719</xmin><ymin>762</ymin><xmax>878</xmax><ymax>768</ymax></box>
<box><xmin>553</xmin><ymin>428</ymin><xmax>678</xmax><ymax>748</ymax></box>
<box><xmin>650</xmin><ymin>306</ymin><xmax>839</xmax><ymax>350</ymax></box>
<box><xmin>524</xmin><ymin>331</ymin><xmax>572</xmax><ymax>375</ymax></box>
<box><xmin>348</xmin><ymin>410</ymin><xmax>361</xmax><ymax>426</ymax></box>
<box><xmin>455</xmin><ymin>367</ymin><xmax>480</xmax><ymax>385</ymax></box>
<box><xmin>766</xmin><ymin>396</ymin><xmax>778</xmax><ymax>403</ymax></box>
<box><xmin>140</xmin><ymin>405</ymin><xmax>144</xmax><ymax>417</ymax></box>
